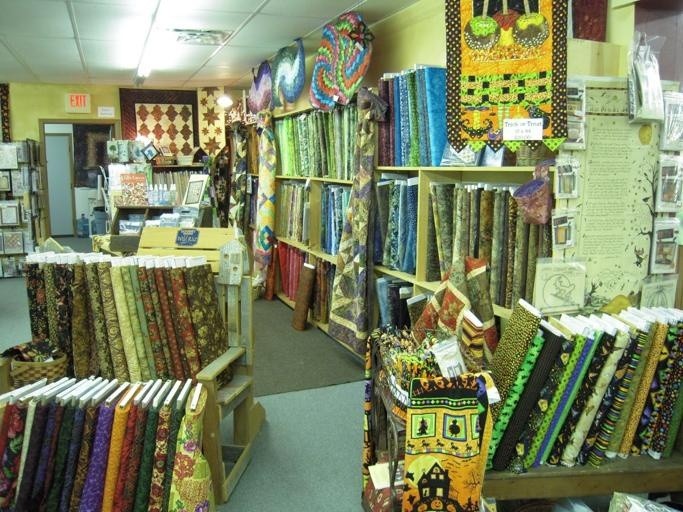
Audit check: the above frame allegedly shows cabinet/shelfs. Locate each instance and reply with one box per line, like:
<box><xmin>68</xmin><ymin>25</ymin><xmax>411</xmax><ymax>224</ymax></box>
<box><xmin>109</xmin><ymin>164</ymin><xmax>218</xmax><ymax>254</ymax></box>
<box><xmin>370</xmin><ymin>165</ymin><xmax>557</xmax><ymax>336</ymax></box>
<box><xmin>244</xmin><ymin>89</ymin><xmax>377</xmax><ymax>360</ymax></box>
<box><xmin>3</xmin><ymin>273</ymin><xmax>267</xmax><ymax>503</ymax></box>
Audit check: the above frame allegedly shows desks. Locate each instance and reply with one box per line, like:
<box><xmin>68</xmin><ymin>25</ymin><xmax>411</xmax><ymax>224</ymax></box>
<box><xmin>376</xmin><ymin>373</ymin><xmax>683</xmax><ymax>512</ymax></box>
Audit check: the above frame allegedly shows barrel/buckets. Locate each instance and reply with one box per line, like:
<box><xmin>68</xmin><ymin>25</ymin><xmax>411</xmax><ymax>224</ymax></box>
<box><xmin>76</xmin><ymin>213</ymin><xmax>89</xmax><ymax>238</ymax></box>
